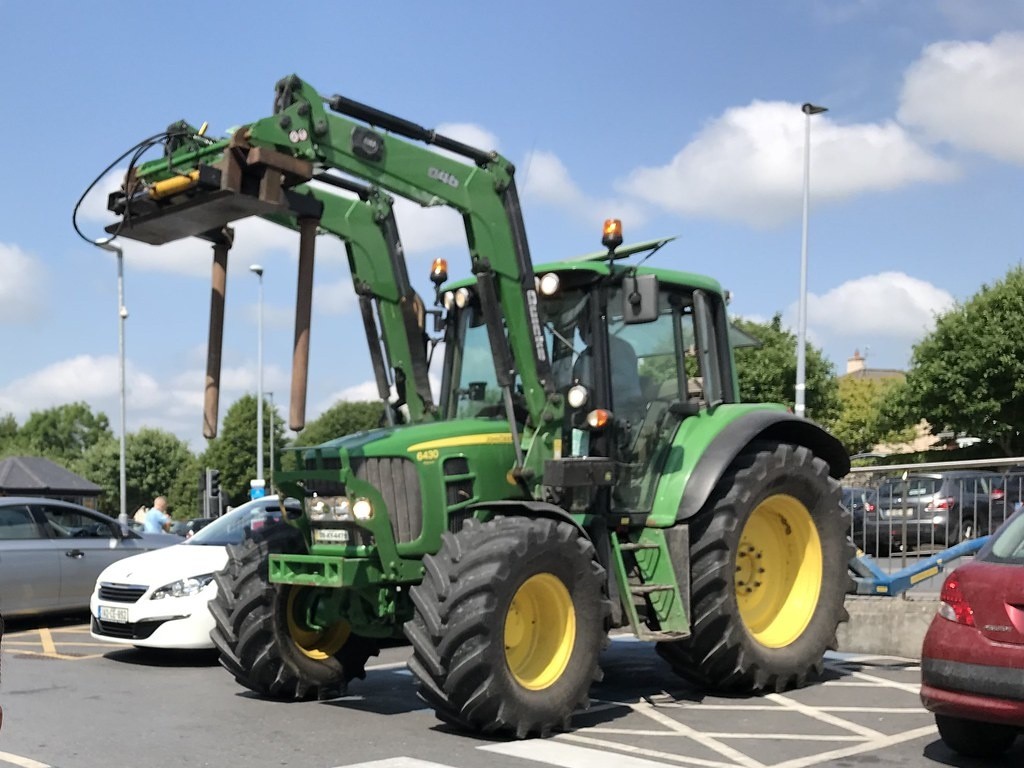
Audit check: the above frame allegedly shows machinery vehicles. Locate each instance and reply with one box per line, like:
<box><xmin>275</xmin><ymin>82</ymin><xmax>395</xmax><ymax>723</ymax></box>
<box><xmin>70</xmin><ymin>74</ymin><xmax>858</xmax><ymax>741</ymax></box>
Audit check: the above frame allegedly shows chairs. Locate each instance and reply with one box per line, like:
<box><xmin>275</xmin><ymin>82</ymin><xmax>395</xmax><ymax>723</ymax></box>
<box><xmin>609</xmin><ymin>373</ymin><xmax>655</xmax><ymax>427</ymax></box>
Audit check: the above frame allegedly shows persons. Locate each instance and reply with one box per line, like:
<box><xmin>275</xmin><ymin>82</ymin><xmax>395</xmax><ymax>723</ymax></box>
<box><xmin>568</xmin><ymin>313</ymin><xmax>643</xmax><ymax>457</ymax></box>
<box><xmin>133</xmin><ymin>495</ymin><xmax>171</xmax><ymax>534</ymax></box>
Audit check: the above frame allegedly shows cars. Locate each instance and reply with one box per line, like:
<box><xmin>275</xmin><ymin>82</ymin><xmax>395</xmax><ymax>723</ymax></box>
<box><xmin>89</xmin><ymin>495</ymin><xmax>301</xmax><ymax>666</ymax></box>
<box><xmin>919</xmin><ymin>505</ymin><xmax>1024</xmax><ymax>757</ymax></box>
<box><xmin>862</xmin><ymin>469</ymin><xmax>1024</xmax><ymax>559</ymax></box>
<box><xmin>841</xmin><ymin>486</ymin><xmax>873</xmax><ymax>538</ymax></box>
<box><xmin>115</xmin><ymin>518</ymin><xmax>217</xmax><ymax>538</ymax></box>
<box><xmin>0</xmin><ymin>497</ymin><xmax>191</xmax><ymax>640</ymax></box>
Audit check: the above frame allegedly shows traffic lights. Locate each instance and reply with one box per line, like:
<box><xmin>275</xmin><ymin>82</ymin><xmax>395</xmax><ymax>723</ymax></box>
<box><xmin>206</xmin><ymin>466</ymin><xmax>222</xmax><ymax>497</ymax></box>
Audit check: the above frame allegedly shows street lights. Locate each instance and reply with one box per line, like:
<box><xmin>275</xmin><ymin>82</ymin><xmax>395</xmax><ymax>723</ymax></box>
<box><xmin>95</xmin><ymin>237</ymin><xmax>131</xmax><ymax>540</ymax></box>
<box><xmin>794</xmin><ymin>102</ymin><xmax>830</xmax><ymax>418</ymax></box>
<box><xmin>262</xmin><ymin>390</ymin><xmax>276</xmax><ymax>495</ymax></box>
<box><xmin>247</xmin><ymin>264</ymin><xmax>267</xmax><ymax>499</ymax></box>
